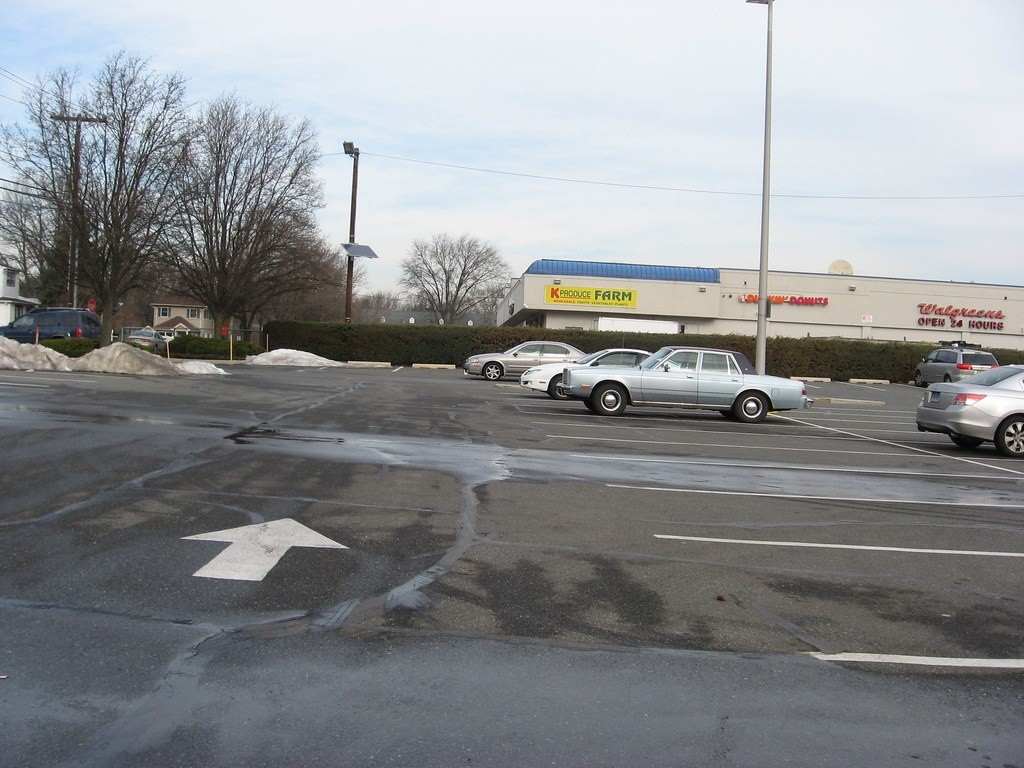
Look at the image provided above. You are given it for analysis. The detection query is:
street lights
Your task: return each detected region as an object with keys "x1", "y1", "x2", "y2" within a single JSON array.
[
  {"x1": 745, "y1": 0, "x2": 778, "y2": 376},
  {"x1": 342, "y1": 139, "x2": 360, "y2": 325}
]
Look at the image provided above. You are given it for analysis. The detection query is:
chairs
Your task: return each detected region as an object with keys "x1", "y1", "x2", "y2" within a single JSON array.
[{"x1": 681, "y1": 361, "x2": 689, "y2": 368}]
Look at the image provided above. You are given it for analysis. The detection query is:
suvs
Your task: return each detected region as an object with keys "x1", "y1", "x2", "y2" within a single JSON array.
[
  {"x1": 913, "y1": 347, "x2": 1001, "y2": 388},
  {"x1": 0, "y1": 307, "x2": 103, "y2": 345}
]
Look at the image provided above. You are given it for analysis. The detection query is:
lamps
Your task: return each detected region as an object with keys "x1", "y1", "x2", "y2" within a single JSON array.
[
  {"x1": 849, "y1": 285, "x2": 855, "y2": 291},
  {"x1": 553, "y1": 280, "x2": 560, "y2": 284},
  {"x1": 699, "y1": 287, "x2": 706, "y2": 292}
]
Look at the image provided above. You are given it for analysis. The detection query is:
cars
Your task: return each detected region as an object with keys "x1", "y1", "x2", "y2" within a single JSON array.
[
  {"x1": 519, "y1": 348, "x2": 689, "y2": 400},
  {"x1": 126, "y1": 330, "x2": 167, "y2": 352},
  {"x1": 560, "y1": 345, "x2": 815, "y2": 423},
  {"x1": 462, "y1": 340, "x2": 601, "y2": 381},
  {"x1": 915, "y1": 364, "x2": 1024, "y2": 458}
]
